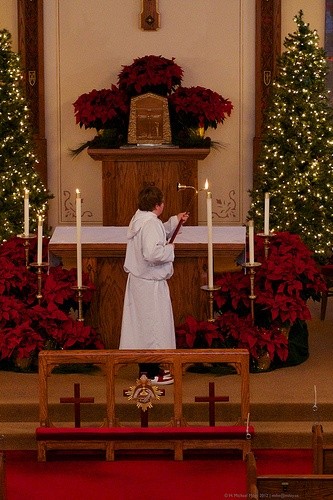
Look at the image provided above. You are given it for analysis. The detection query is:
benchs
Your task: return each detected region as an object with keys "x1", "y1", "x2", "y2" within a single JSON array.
[{"x1": 35, "y1": 425, "x2": 254, "y2": 463}]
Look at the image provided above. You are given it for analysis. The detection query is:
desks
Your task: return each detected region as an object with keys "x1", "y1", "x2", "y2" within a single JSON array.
[{"x1": 48, "y1": 226, "x2": 246, "y2": 349}]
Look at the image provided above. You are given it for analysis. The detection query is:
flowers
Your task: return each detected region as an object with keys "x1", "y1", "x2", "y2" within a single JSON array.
[
  {"x1": 0, "y1": 233, "x2": 105, "y2": 360},
  {"x1": 174, "y1": 230, "x2": 327, "y2": 362},
  {"x1": 72, "y1": 55, "x2": 234, "y2": 132}
]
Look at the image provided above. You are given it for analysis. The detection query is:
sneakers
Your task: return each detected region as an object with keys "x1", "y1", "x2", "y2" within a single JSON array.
[
  {"x1": 158, "y1": 369, "x2": 171, "y2": 376},
  {"x1": 150, "y1": 375, "x2": 174, "y2": 384}
]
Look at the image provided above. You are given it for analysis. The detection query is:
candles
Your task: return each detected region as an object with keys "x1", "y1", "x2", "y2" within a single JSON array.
[
  {"x1": 37, "y1": 221, "x2": 42, "y2": 265},
  {"x1": 76, "y1": 193, "x2": 82, "y2": 288},
  {"x1": 24, "y1": 194, "x2": 29, "y2": 237},
  {"x1": 264, "y1": 192, "x2": 269, "y2": 236},
  {"x1": 207, "y1": 191, "x2": 213, "y2": 288},
  {"x1": 249, "y1": 220, "x2": 254, "y2": 265}
]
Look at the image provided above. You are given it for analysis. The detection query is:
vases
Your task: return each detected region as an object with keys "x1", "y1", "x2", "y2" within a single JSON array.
[
  {"x1": 254, "y1": 351, "x2": 272, "y2": 371},
  {"x1": 13, "y1": 350, "x2": 34, "y2": 373}
]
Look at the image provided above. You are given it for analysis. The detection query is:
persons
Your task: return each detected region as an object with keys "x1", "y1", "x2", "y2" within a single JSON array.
[{"x1": 119, "y1": 187, "x2": 190, "y2": 385}]
